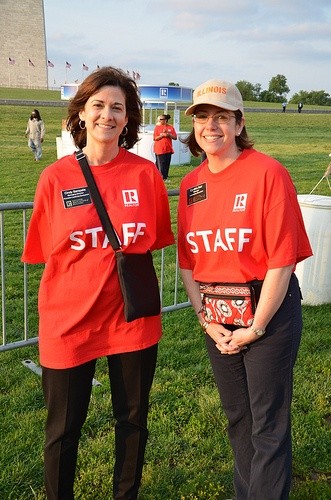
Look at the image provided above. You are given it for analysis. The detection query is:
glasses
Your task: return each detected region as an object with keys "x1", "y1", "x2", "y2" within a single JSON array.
[{"x1": 193, "y1": 113, "x2": 237, "y2": 124}]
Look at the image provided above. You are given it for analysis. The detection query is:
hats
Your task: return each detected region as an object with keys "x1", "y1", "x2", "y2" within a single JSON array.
[{"x1": 185, "y1": 79, "x2": 245, "y2": 116}]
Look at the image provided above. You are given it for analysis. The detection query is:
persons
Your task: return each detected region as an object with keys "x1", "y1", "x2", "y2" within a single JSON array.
[
  {"x1": 282, "y1": 101, "x2": 286, "y2": 112},
  {"x1": 298, "y1": 101, "x2": 303, "y2": 113},
  {"x1": 153, "y1": 114, "x2": 177, "y2": 181},
  {"x1": 175, "y1": 79, "x2": 314, "y2": 500},
  {"x1": 21, "y1": 66, "x2": 176, "y2": 500},
  {"x1": 24, "y1": 109, "x2": 44, "y2": 162}
]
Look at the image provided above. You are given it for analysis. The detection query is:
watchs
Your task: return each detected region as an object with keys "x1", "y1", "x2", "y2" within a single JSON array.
[{"x1": 251, "y1": 327, "x2": 265, "y2": 336}]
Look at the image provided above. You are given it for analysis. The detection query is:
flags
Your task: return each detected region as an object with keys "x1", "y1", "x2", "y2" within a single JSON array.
[
  {"x1": 29, "y1": 59, "x2": 34, "y2": 66},
  {"x1": 48, "y1": 60, "x2": 54, "y2": 68},
  {"x1": 66, "y1": 62, "x2": 72, "y2": 69},
  {"x1": 127, "y1": 70, "x2": 141, "y2": 79},
  {"x1": 9, "y1": 58, "x2": 15, "y2": 65},
  {"x1": 83, "y1": 64, "x2": 88, "y2": 71}
]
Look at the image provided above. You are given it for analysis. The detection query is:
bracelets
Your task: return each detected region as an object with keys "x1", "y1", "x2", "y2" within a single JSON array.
[{"x1": 202, "y1": 322, "x2": 209, "y2": 333}]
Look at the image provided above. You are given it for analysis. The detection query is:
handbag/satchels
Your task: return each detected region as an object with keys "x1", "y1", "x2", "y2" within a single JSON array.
[
  {"x1": 116, "y1": 250, "x2": 160, "y2": 322},
  {"x1": 198, "y1": 281, "x2": 258, "y2": 327}
]
